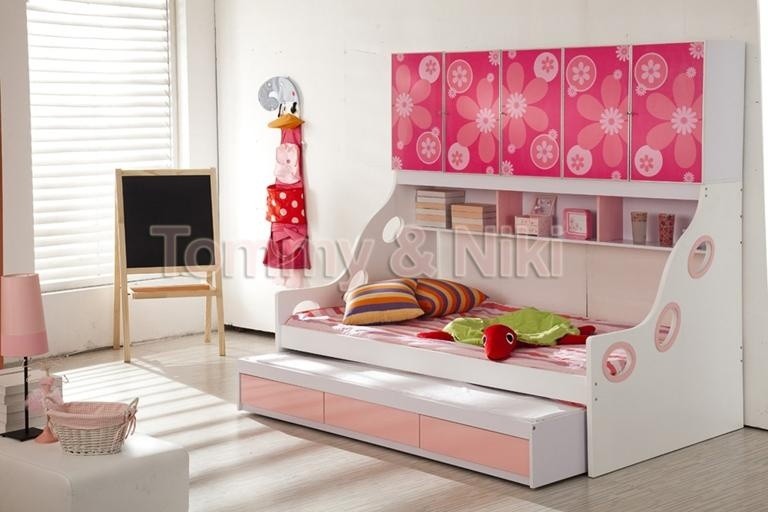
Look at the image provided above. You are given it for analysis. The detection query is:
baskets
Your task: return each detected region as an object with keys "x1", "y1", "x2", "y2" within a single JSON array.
[{"x1": 45, "y1": 397, "x2": 140, "y2": 454}]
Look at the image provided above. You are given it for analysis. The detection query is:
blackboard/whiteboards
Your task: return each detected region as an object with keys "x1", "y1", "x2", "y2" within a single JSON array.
[{"x1": 115, "y1": 168, "x2": 221, "y2": 274}]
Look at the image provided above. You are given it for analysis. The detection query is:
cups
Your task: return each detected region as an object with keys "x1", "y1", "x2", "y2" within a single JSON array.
[
  {"x1": 631, "y1": 211, "x2": 648, "y2": 243},
  {"x1": 658, "y1": 213, "x2": 676, "y2": 246}
]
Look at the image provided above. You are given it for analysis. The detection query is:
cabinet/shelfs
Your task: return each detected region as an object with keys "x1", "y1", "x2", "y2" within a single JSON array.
[{"x1": 390, "y1": 41, "x2": 744, "y2": 252}]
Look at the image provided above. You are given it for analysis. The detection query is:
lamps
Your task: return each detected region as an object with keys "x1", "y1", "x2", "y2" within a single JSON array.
[{"x1": 0, "y1": 270, "x2": 51, "y2": 442}]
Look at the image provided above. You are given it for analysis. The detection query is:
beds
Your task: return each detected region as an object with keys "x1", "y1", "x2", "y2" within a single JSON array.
[{"x1": 276, "y1": 283, "x2": 743, "y2": 477}]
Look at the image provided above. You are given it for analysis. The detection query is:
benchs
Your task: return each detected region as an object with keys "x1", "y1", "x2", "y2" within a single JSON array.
[{"x1": 236, "y1": 352, "x2": 585, "y2": 491}]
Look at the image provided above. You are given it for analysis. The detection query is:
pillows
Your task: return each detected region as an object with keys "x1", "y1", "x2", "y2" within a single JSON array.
[{"x1": 342, "y1": 273, "x2": 490, "y2": 326}]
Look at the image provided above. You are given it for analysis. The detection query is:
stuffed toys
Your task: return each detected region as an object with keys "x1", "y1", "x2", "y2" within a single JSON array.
[{"x1": 417, "y1": 307, "x2": 596, "y2": 361}]
